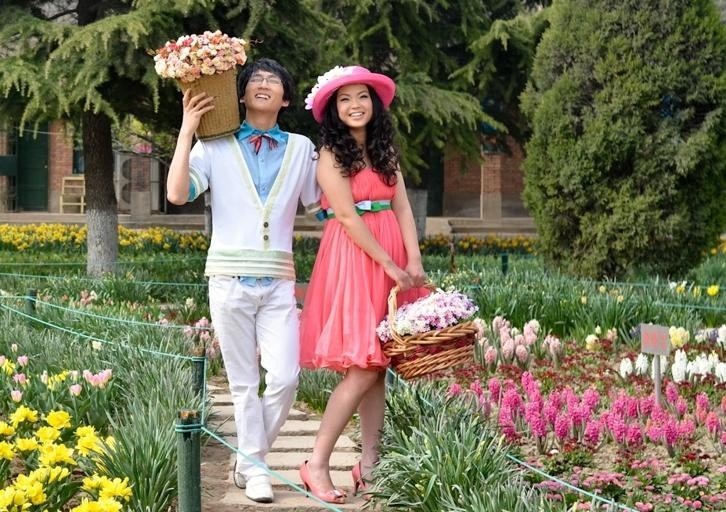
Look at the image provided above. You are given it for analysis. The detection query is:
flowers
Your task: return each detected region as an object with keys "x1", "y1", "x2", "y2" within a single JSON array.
[
  {"x1": 149, "y1": 27, "x2": 248, "y2": 85},
  {"x1": 374, "y1": 288, "x2": 481, "y2": 348}
]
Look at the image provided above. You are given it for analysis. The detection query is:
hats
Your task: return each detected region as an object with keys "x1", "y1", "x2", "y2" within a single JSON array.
[{"x1": 305, "y1": 65, "x2": 396, "y2": 123}]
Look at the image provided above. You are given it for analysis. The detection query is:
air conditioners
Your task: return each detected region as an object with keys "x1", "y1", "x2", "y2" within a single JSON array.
[{"x1": 115, "y1": 150, "x2": 160, "y2": 214}]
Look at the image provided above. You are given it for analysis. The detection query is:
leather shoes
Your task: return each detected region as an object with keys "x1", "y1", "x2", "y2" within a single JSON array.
[
  {"x1": 233, "y1": 461, "x2": 245, "y2": 489},
  {"x1": 245, "y1": 475, "x2": 273, "y2": 503}
]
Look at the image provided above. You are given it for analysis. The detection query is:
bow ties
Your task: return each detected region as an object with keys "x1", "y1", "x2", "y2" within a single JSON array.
[{"x1": 250, "y1": 133, "x2": 276, "y2": 154}]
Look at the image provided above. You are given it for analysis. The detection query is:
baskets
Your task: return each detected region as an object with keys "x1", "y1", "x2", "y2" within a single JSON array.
[
  {"x1": 382, "y1": 279, "x2": 478, "y2": 380},
  {"x1": 175, "y1": 67, "x2": 242, "y2": 142}
]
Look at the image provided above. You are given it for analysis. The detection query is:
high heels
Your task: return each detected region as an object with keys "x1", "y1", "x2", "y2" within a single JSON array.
[
  {"x1": 299, "y1": 460, "x2": 347, "y2": 504},
  {"x1": 351, "y1": 460, "x2": 370, "y2": 501}
]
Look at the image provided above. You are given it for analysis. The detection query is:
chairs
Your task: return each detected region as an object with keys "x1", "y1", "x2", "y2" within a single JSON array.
[{"x1": 59, "y1": 175, "x2": 86, "y2": 214}]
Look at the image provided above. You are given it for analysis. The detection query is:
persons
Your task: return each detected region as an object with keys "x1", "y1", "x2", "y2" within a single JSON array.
[
  {"x1": 300, "y1": 64, "x2": 427, "y2": 505},
  {"x1": 165, "y1": 58, "x2": 327, "y2": 502}
]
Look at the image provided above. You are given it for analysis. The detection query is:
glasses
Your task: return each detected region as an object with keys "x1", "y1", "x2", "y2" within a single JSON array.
[{"x1": 248, "y1": 76, "x2": 283, "y2": 88}]
